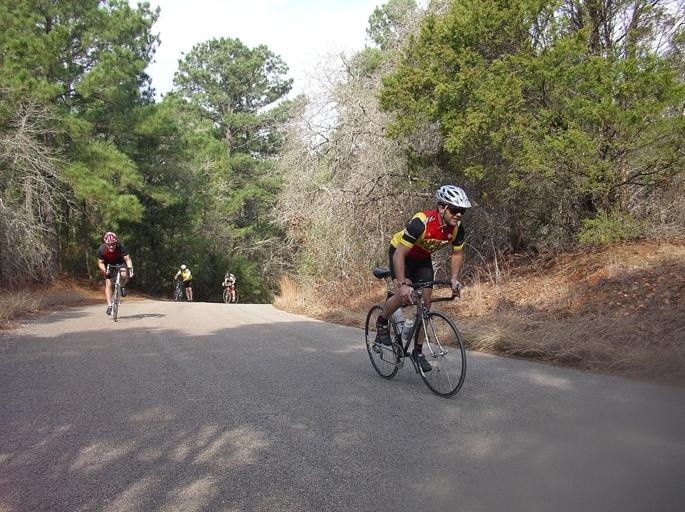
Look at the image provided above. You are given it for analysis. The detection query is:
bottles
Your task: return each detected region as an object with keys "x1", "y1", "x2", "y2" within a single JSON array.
[
  {"x1": 393, "y1": 308, "x2": 404, "y2": 335},
  {"x1": 402, "y1": 317, "x2": 414, "y2": 339}
]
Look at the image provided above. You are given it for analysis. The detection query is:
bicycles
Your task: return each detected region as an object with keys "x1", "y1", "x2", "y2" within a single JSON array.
[
  {"x1": 102, "y1": 262, "x2": 134, "y2": 324},
  {"x1": 362, "y1": 263, "x2": 470, "y2": 399},
  {"x1": 220, "y1": 281, "x2": 240, "y2": 304},
  {"x1": 170, "y1": 278, "x2": 184, "y2": 302}
]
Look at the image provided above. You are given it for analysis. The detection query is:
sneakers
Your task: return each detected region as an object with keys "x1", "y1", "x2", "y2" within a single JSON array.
[
  {"x1": 375, "y1": 318, "x2": 392, "y2": 346},
  {"x1": 106, "y1": 306, "x2": 112, "y2": 315},
  {"x1": 412, "y1": 348, "x2": 432, "y2": 372},
  {"x1": 120, "y1": 286, "x2": 126, "y2": 297}
]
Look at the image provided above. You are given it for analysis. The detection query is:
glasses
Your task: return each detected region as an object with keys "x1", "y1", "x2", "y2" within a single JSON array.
[{"x1": 447, "y1": 206, "x2": 467, "y2": 215}]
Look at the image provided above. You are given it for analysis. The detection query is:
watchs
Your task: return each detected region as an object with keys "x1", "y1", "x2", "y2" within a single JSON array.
[{"x1": 396, "y1": 281, "x2": 407, "y2": 289}]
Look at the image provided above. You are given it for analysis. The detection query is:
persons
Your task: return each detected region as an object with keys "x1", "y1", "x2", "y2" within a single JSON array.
[
  {"x1": 174, "y1": 263, "x2": 193, "y2": 302},
  {"x1": 221, "y1": 272, "x2": 236, "y2": 303},
  {"x1": 375, "y1": 184, "x2": 471, "y2": 373},
  {"x1": 96, "y1": 231, "x2": 135, "y2": 316}
]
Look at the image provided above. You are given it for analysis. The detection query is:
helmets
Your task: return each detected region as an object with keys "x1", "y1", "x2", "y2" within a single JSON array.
[
  {"x1": 103, "y1": 232, "x2": 117, "y2": 245},
  {"x1": 181, "y1": 264, "x2": 186, "y2": 270},
  {"x1": 435, "y1": 185, "x2": 472, "y2": 209}
]
